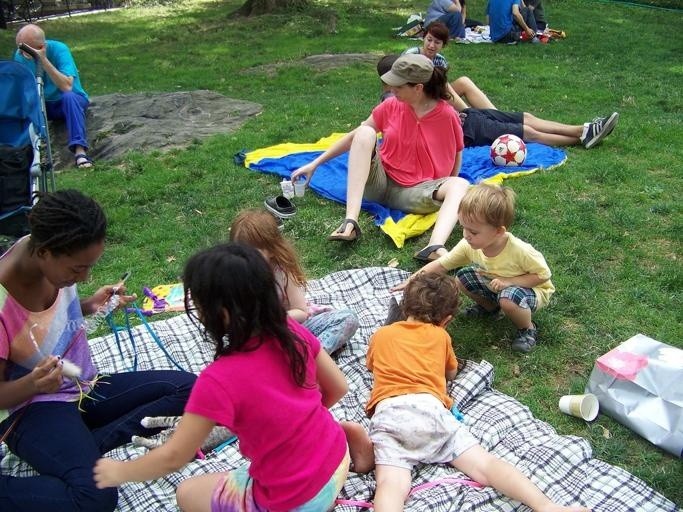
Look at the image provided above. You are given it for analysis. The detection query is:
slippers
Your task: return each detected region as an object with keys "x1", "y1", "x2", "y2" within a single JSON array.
[
  {"x1": 328, "y1": 219, "x2": 361, "y2": 240},
  {"x1": 413, "y1": 245, "x2": 449, "y2": 261}
]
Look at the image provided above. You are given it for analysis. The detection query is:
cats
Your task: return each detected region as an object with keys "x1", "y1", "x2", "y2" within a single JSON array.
[{"x1": 130, "y1": 416, "x2": 233, "y2": 454}]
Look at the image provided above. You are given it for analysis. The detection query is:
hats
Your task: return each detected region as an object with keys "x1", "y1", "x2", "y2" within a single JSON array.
[{"x1": 380, "y1": 54, "x2": 434, "y2": 87}]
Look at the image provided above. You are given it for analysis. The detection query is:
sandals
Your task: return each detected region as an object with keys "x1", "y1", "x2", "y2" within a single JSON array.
[{"x1": 73, "y1": 153, "x2": 94, "y2": 170}]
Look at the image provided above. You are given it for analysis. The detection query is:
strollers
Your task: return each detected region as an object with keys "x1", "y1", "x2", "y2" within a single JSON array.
[{"x1": 0, "y1": 42, "x2": 56, "y2": 238}]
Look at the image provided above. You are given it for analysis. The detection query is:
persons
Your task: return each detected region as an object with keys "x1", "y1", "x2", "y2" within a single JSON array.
[
  {"x1": 400, "y1": 21, "x2": 497, "y2": 113},
  {"x1": 92, "y1": 240, "x2": 375, "y2": 512},
  {"x1": 424, "y1": 0, "x2": 546, "y2": 45},
  {"x1": 14, "y1": 24, "x2": 93, "y2": 170},
  {"x1": 229, "y1": 208, "x2": 359, "y2": 355},
  {"x1": 0, "y1": 188, "x2": 198, "y2": 512},
  {"x1": 290, "y1": 55, "x2": 470, "y2": 262},
  {"x1": 390, "y1": 184, "x2": 556, "y2": 353},
  {"x1": 365, "y1": 270, "x2": 592, "y2": 512},
  {"x1": 377, "y1": 56, "x2": 619, "y2": 149}
]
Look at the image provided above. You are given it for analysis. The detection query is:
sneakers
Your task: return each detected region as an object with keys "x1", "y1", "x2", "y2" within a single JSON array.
[
  {"x1": 457, "y1": 305, "x2": 504, "y2": 324},
  {"x1": 579, "y1": 113, "x2": 618, "y2": 149},
  {"x1": 511, "y1": 321, "x2": 538, "y2": 353},
  {"x1": 264, "y1": 196, "x2": 296, "y2": 218}
]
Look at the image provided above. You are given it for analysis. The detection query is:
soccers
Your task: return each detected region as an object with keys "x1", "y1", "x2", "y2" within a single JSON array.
[{"x1": 491, "y1": 134, "x2": 526, "y2": 167}]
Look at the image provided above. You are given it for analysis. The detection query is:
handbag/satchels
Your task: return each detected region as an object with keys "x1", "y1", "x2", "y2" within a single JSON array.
[{"x1": 584, "y1": 334, "x2": 683, "y2": 457}]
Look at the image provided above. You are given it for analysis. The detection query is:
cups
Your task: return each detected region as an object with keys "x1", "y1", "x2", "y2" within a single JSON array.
[
  {"x1": 278, "y1": 180, "x2": 295, "y2": 197},
  {"x1": 558, "y1": 393, "x2": 602, "y2": 423},
  {"x1": 292, "y1": 181, "x2": 306, "y2": 197}
]
